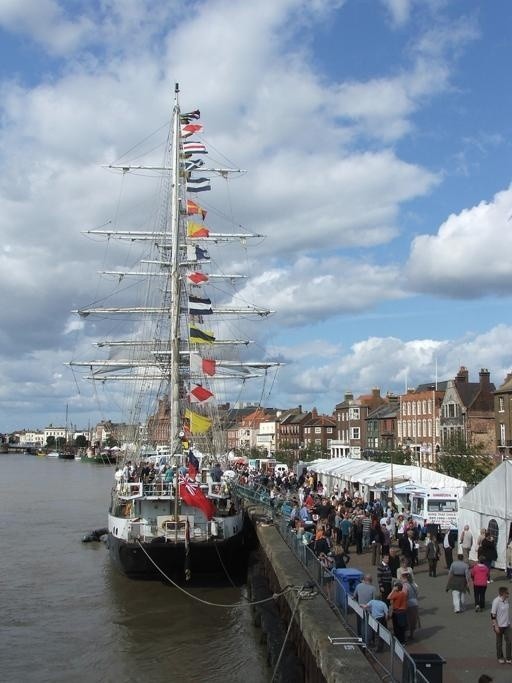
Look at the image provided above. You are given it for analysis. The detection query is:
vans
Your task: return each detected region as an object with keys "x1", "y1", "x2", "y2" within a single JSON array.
[{"x1": 408, "y1": 492, "x2": 458, "y2": 540}]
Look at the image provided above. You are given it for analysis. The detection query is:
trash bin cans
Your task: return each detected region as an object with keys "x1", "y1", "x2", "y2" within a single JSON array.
[
  {"x1": 403, "y1": 653, "x2": 446, "y2": 683},
  {"x1": 333, "y1": 568, "x2": 364, "y2": 614},
  {"x1": 427, "y1": 524, "x2": 441, "y2": 541}
]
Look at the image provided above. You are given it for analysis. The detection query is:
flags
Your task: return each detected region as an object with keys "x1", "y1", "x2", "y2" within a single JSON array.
[{"x1": 177, "y1": 106, "x2": 221, "y2": 522}]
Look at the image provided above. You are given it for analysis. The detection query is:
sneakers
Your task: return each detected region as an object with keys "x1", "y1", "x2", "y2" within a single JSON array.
[
  {"x1": 496, "y1": 657, "x2": 512, "y2": 664},
  {"x1": 474, "y1": 604, "x2": 480, "y2": 613}
]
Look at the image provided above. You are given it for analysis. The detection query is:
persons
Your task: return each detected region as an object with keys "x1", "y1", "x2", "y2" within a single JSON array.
[
  {"x1": 109, "y1": 449, "x2": 324, "y2": 496},
  {"x1": 277, "y1": 482, "x2": 511, "y2": 653},
  {"x1": 489, "y1": 585, "x2": 511, "y2": 665}
]
